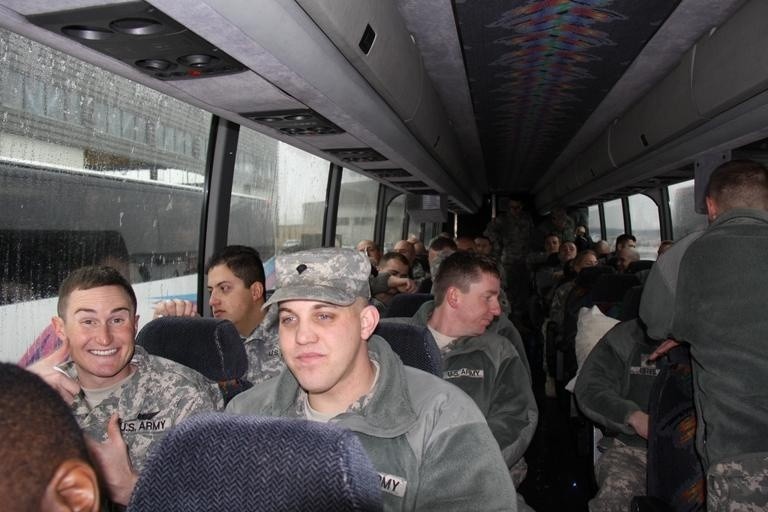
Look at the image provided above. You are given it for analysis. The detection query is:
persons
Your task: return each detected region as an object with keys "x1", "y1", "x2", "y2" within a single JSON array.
[{"x1": 2, "y1": 159, "x2": 767, "y2": 512}]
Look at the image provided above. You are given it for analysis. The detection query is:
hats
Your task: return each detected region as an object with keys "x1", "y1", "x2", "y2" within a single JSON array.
[{"x1": 257, "y1": 244, "x2": 373, "y2": 313}]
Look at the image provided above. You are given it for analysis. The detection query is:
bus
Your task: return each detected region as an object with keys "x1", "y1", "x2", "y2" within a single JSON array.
[{"x1": 0, "y1": 26, "x2": 278, "y2": 367}]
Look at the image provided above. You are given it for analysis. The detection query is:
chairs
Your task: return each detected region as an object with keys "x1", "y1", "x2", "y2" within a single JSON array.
[{"x1": 128, "y1": 245, "x2": 708, "y2": 512}]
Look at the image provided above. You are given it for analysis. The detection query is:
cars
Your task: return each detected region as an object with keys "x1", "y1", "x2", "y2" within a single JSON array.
[
  {"x1": 341, "y1": 241, "x2": 358, "y2": 252},
  {"x1": 590, "y1": 237, "x2": 661, "y2": 248},
  {"x1": 382, "y1": 240, "x2": 395, "y2": 253},
  {"x1": 282, "y1": 240, "x2": 308, "y2": 253}
]
